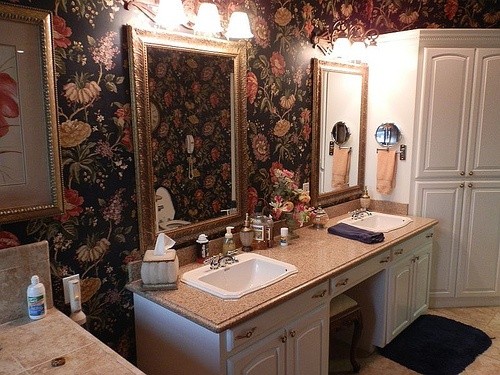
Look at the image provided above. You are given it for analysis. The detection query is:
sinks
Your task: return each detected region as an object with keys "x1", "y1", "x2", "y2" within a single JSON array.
[
  {"x1": 337, "y1": 211, "x2": 413, "y2": 233},
  {"x1": 179, "y1": 251, "x2": 299, "y2": 299}
]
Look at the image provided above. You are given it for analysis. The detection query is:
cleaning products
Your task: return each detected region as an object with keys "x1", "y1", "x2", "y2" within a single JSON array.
[
  {"x1": 195, "y1": 233, "x2": 210, "y2": 264},
  {"x1": 26, "y1": 275, "x2": 47, "y2": 320},
  {"x1": 221, "y1": 226, "x2": 236, "y2": 257}
]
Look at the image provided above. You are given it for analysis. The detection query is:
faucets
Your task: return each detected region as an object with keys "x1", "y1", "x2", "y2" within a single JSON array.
[
  {"x1": 348, "y1": 207, "x2": 372, "y2": 220},
  {"x1": 203, "y1": 248, "x2": 240, "y2": 271}
]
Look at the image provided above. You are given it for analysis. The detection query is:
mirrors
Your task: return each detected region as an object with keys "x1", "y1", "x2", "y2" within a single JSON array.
[
  {"x1": 127, "y1": 23, "x2": 249, "y2": 256},
  {"x1": 310, "y1": 58, "x2": 369, "y2": 209},
  {"x1": 375, "y1": 123, "x2": 405, "y2": 161},
  {"x1": 329, "y1": 121, "x2": 353, "y2": 156}
]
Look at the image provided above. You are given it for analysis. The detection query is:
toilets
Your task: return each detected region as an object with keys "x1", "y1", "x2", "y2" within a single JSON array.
[{"x1": 155, "y1": 186, "x2": 191, "y2": 232}]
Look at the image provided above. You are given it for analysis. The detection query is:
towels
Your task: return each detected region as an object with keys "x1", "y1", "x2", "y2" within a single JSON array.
[
  {"x1": 331, "y1": 148, "x2": 351, "y2": 190},
  {"x1": 375, "y1": 150, "x2": 398, "y2": 196},
  {"x1": 325, "y1": 222, "x2": 386, "y2": 245}
]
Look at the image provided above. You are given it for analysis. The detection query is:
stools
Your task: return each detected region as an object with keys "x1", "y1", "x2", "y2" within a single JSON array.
[{"x1": 329, "y1": 294, "x2": 363, "y2": 374}]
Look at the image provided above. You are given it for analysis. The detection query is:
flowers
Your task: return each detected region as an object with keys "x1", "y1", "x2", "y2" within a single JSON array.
[{"x1": 258, "y1": 162, "x2": 316, "y2": 226}]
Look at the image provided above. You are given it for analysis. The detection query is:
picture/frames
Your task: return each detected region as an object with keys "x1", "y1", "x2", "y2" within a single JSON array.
[{"x1": 0, "y1": 2, "x2": 66, "y2": 224}]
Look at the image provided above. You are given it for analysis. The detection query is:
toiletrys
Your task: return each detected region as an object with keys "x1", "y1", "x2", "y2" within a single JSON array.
[
  {"x1": 239, "y1": 212, "x2": 254, "y2": 251},
  {"x1": 359, "y1": 185, "x2": 371, "y2": 211},
  {"x1": 263, "y1": 209, "x2": 274, "y2": 248},
  {"x1": 250, "y1": 198, "x2": 267, "y2": 250}
]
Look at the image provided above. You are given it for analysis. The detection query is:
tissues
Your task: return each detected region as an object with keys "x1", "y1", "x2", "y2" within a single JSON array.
[{"x1": 139, "y1": 232, "x2": 180, "y2": 292}]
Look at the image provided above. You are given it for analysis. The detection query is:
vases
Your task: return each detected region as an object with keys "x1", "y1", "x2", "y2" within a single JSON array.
[{"x1": 284, "y1": 214, "x2": 299, "y2": 240}]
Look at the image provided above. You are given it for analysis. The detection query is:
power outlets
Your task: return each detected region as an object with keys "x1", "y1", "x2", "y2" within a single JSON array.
[{"x1": 61, "y1": 274, "x2": 81, "y2": 305}]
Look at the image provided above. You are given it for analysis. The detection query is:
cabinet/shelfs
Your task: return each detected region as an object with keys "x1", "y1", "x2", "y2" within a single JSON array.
[
  {"x1": 133, "y1": 226, "x2": 434, "y2": 375},
  {"x1": 367, "y1": 28, "x2": 500, "y2": 308}
]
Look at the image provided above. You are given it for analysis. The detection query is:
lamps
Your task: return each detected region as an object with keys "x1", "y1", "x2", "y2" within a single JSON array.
[
  {"x1": 312, "y1": 35, "x2": 367, "y2": 61},
  {"x1": 125, "y1": 0, "x2": 254, "y2": 39}
]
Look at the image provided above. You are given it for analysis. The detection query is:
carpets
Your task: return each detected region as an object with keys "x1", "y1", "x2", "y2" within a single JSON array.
[{"x1": 375, "y1": 315, "x2": 492, "y2": 375}]
